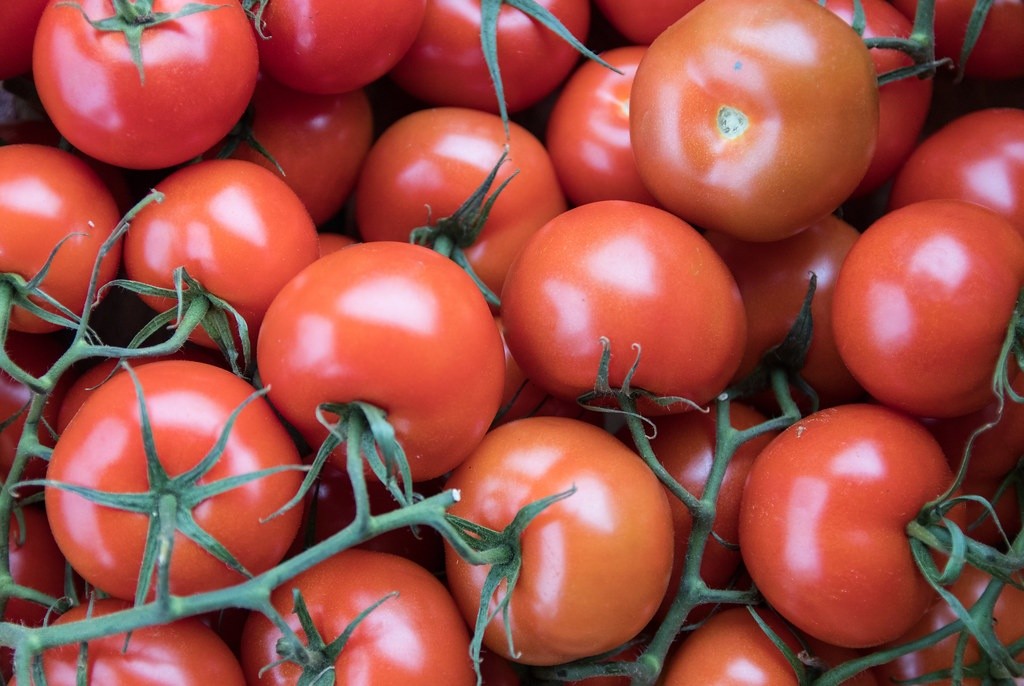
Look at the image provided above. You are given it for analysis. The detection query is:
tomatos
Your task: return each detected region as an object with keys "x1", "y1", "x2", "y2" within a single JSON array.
[{"x1": 0, "y1": 0, "x2": 1024, "y2": 686}]
